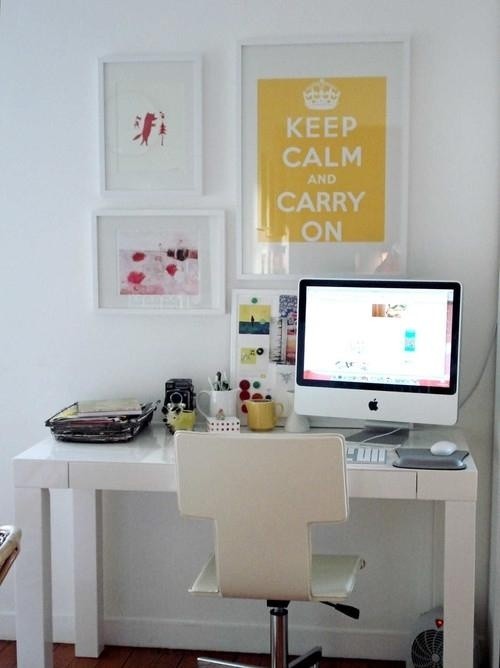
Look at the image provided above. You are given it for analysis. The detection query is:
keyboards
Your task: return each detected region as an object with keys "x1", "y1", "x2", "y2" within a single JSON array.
[{"x1": 346, "y1": 447, "x2": 388, "y2": 464}]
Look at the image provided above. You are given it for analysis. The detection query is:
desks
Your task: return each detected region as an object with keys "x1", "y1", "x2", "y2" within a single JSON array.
[{"x1": 13, "y1": 420, "x2": 480, "y2": 666}]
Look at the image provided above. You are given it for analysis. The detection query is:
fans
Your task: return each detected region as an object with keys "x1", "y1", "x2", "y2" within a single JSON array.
[{"x1": 402, "y1": 601, "x2": 444, "y2": 667}]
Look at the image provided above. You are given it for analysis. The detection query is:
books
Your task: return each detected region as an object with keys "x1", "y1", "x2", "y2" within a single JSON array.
[{"x1": 50, "y1": 397, "x2": 160, "y2": 440}]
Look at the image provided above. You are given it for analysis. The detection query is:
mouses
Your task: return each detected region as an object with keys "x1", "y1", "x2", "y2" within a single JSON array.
[{"x1": 431, "y1": 440, "x2": 457, "y2": 456}]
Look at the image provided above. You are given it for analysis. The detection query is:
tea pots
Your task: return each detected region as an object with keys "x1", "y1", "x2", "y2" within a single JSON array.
[
  {"x1": 195, "y1": 387, "x2": 242, "y2": 419},
  {"x1": 244, "y1": 398, "x2": 283, "y2": 431}
]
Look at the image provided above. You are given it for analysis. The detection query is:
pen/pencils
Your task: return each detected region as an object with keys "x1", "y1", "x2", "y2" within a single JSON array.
[{"x1": 207, "y1": 371, "x2": 232, "y2": 391}]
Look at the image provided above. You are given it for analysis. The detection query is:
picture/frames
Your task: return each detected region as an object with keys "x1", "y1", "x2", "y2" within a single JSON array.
[
  {"x1": 98, "y1": 58, "x2": 204, "y2": 202},
  {"x1": 87, "y1": 206, "x2": 225, "y2": 318}
]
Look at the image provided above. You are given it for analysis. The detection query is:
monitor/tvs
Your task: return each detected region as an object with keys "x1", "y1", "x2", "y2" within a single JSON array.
[{"x1": 297, "y1": 278, "x2": 462, "y2": 449}]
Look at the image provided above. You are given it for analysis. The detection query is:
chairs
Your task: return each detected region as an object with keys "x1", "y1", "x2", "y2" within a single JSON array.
[{"x1": 170, "y1": 426, "x2": 371, "y2": 666}]
